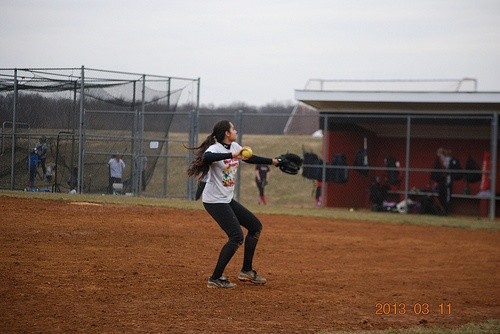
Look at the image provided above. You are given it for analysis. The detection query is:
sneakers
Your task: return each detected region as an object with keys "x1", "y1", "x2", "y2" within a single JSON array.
[
  {"x1": 207, "y1": 277, "x2": 235, "y2": 287},
  {"x1": 238, "y1": 272, "x2": 267, "y2": 285}
]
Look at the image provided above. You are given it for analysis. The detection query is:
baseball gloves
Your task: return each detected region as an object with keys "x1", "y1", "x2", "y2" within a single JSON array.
[{"x1": 274, "y1": 153, "x2": 303, "y2": 175}]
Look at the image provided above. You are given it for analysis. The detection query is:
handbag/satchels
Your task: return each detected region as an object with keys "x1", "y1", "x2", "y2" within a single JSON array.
[{"x1": 354, "y1": 150, "x2": 482, "y2": 212}]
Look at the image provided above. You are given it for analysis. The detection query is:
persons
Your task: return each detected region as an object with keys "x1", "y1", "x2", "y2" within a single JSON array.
[
  {"x1": 26, "y1": 136, "x2": 56, "y2": 190},
  {"x1": 311, "y1": 178, "x2": 323, "y2": 206},
  {"x1": 107, "y1": 152, "x2": 125, "y2": 193},
  {"x1": 369, "y1": 176, "x2": 393, "y2": 212},
  {"x1": 68, "y1": 168, "x2": 78, "y2": 191},
  {"x1": 195, "y1": 173, "x2": 209, "y2": 200},
  {"x1": 432, "y1": 147, "x2": 459, "y2": 204},
  {"x1": 255, "y1": 164, "x2": 270, "y2": 204},
  {"x1": 185, "y1": 119, "x2": 303, "y2": 288}
]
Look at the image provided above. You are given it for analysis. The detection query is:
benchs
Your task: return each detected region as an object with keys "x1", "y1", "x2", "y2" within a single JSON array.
[{"x1": 386, "y1": 189, "x2": 500, "y2": 215}]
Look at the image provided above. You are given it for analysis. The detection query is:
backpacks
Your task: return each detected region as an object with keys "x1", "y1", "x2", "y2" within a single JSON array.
[
  {"x1": 299, "y1": 152, "x2": 321, "y2": 179},
  {"x1": 330, "y1": 157, "x2": 348, "y2": 184}
]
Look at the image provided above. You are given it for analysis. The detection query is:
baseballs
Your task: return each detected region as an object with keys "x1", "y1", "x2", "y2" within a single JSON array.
[{"x1": 242, "y1": 147, "x2": 253, "y2": 158}]
